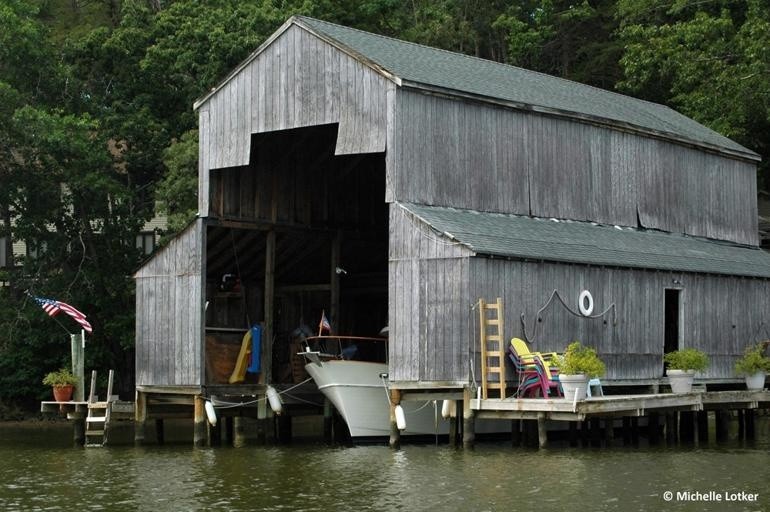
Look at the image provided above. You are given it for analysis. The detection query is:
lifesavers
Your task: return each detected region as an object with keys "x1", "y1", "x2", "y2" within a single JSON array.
[{"x1": 578, "y1": 289, "x2": 595, "y2": 315}]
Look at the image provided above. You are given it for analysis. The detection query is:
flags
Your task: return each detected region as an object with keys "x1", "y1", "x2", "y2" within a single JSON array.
[{"x1": 27, "y1": 293, "x2": 94, "y2": 335}]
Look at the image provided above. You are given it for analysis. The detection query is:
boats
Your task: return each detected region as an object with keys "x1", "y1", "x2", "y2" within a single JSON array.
[{"x1": 296, "y1": 307, "x2": 693, "y2": 439}]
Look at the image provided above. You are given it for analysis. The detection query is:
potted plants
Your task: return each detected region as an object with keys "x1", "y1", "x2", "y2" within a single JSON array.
[
  {"x1": 664, "y1": 349, "x2": 709, "y2": 396},
  {"x1": 733, "y1": 344, "x2": 770, "y2": 390},
  {"x1": 553, "y1": 341, "x2": 605, "y2": 399},
  {"x1": 43, "y1": 368, "x2": 78, "y2": 402}
]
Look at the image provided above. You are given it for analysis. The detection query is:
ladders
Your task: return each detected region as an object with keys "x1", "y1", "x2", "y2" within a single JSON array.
[
  {"x1": 83, "y1": 370, "x2": 114, "y2": 448},
  {"x1": 479, "y1": 298, "x2": 507, "y2": 400}
]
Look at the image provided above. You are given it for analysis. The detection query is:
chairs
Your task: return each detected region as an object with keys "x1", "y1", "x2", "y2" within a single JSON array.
[{"x1": 508, "y1": 338, "x2": 563, "y2": 398}]
